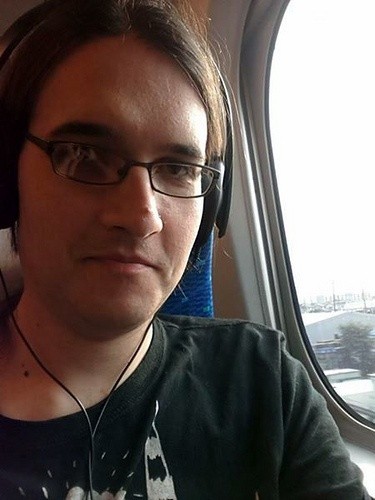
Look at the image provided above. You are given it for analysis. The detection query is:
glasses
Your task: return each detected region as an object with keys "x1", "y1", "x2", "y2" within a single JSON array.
[{"x1": 21, "y1": 132, "x2": 222, "y2": 200}]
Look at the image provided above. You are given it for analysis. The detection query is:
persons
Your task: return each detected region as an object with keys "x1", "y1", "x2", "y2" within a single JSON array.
[{"x1": 0, "y1": 0, "x2": 375, "y2": 500}]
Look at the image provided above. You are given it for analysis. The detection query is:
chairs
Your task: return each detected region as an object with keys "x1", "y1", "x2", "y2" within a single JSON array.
[{"x1": 0, "y1": 227, "x2": 214, "y2": 316}]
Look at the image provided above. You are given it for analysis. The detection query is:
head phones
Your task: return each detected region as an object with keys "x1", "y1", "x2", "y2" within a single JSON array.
[{"x1": 0, "y1": 0, "x2": 233, "y2": 250}]
